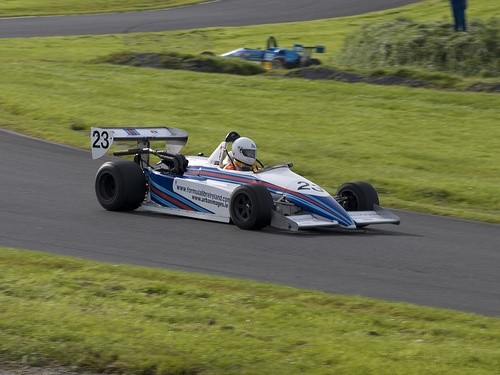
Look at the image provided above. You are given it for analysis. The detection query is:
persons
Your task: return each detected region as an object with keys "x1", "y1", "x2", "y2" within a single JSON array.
[
  {"x1": 223, "y1": 137, "x2": 260, "y2": 172},
  {"x1": 448, "y1": 0, "x2": 467, "y2": 33}
]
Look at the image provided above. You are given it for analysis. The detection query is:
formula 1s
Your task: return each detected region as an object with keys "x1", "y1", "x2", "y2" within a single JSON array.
[
  {"x1": 199, "y1": 35, "x2": 328, "y2": 70},
  {"x1": 90, "y1": 125, "x2": 400, "y2": 235}
]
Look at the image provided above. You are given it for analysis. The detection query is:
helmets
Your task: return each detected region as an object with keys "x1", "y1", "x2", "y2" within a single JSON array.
[{"x1": 232, "y1": 136, "x2": 256, "y2": 166}]
